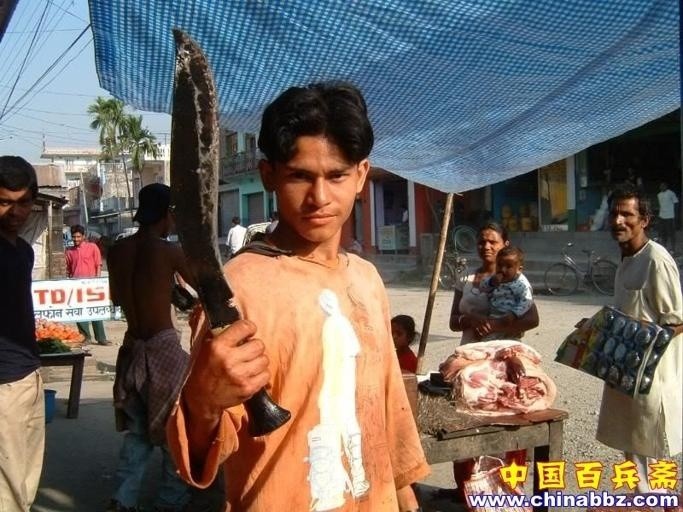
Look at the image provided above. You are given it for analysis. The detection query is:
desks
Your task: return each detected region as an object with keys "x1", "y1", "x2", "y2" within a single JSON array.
[
  {"x1": 416, "y1": 407, "x2": 572, "y2": 512},
  {"x1": 38, "y1": 347, "x2": 92, "y2": 418}
]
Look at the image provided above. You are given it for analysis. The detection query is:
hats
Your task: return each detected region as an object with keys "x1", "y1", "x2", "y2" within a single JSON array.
[{"x1": 133, "y1": 183, "x2": 170, "y2": 226}]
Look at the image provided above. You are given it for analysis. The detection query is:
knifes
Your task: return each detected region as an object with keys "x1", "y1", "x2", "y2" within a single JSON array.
[{"x1": 169, "y1": 26, "x2": 295, "y2": 442}]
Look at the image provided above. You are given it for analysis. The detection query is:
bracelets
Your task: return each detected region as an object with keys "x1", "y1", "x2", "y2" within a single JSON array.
[{"x1": 456, "y1": 314, "x2": 467, "y2": 323}]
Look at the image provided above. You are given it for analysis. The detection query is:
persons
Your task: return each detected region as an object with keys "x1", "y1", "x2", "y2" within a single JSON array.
[
  {"x1": 398, "y1": 205, "x2": 409, "y2": 225},
  {"x1": 163, "y1": 81, "x2": 433, "y2": 512},
  {"x1": 64, "y1": 224, "x2": 112, "y2": 346},
  {"x1": 0, "y1": 155, "x2": 47, "y2": 512},
  {"x1": 654, "y1": 181, "x2": 680, "y2": 256},
  {"x1": 221, "y1": 217, "x2": 246, "y2": 260},
  {"x1": 478, "y1": 244, "x2": 533, "y2": 344},
  {"x1": 447, "y1": 221, "x2": 540, "y2": 348},
  {"x1": 625, "y1": 166, "x2": 644, "y2": 194},
  {"x1": 105, "y1": 182, "x2": 198, "y2": 511},
  {"x1": 263, "y1": 211, "x2": 280, "y2": 234},
  {"x1": 572, "y1": 180, "x2": 683, "y2": 512},
  {"x1": 345, "y1": 236, "x2": 362, "y2": 256},
  {"x1": 387, "y1": 314, "x2": 419, "y2": 374}
]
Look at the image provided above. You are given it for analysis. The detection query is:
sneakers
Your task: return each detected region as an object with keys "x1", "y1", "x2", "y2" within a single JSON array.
[{"x1": 83, "y1": 337, "x2": 112, "y2": 345}]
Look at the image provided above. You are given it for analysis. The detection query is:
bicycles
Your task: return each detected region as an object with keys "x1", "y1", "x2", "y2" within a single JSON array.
[
  {"x1": 543, "y1": 242, "x2": 619, "y2": 297},
  {"x1": 438, "y1": 249, "x2": 470, "y2": 291}
]
